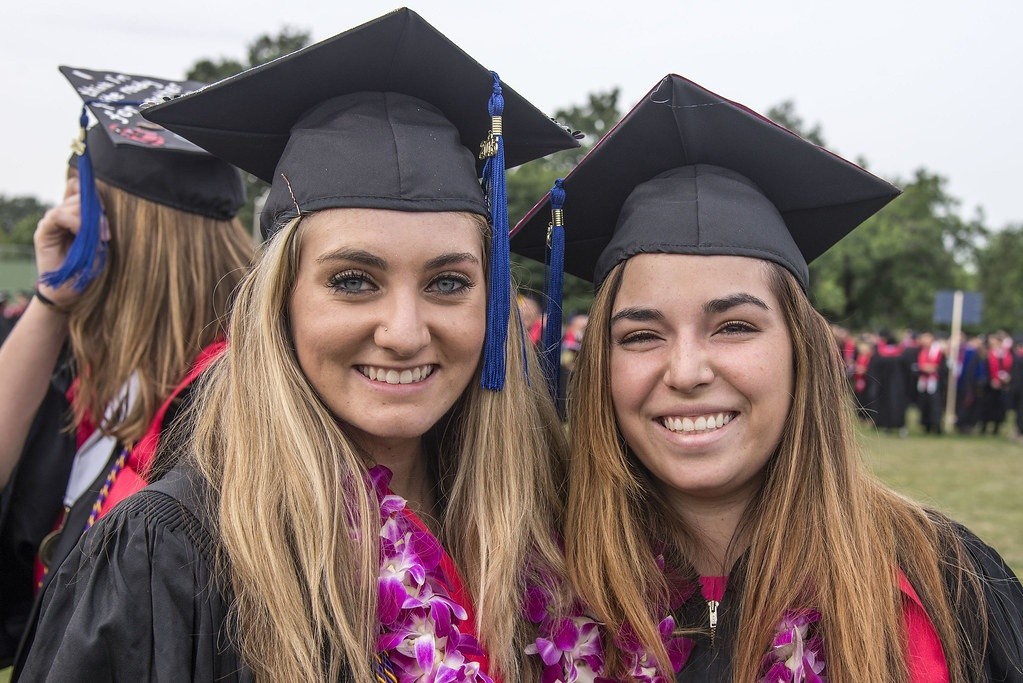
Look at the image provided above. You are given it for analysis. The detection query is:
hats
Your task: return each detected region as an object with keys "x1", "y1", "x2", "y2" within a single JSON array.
[
  {"x1": 40, "y1": 64, "x2": 246, "y2": 292},
  {"x1": 139, "y1": 6, "x2": 585, "y2": 392},
  {"x1": 508, "y1": 73, "x2": 904, "y2": 417}
]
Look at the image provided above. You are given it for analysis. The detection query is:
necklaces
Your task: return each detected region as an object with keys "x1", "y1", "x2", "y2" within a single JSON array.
[
  {"x1": 611, "y1": 530, "x2": 827, "y2": 683},
  {"x1": 359, "y1": 463, "x2": 604, "y2": 683}
]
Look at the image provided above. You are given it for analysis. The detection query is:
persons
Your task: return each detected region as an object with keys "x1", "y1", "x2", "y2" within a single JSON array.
[
  {"x1": 516, "y1": 280, "x2": 590, "y2": 422},
  {"x1": 507, "y1": 73, "x2": 1023, "y2": 683},
  {"x1": 827, "y1": 316, "x2": 1023, "y2": 436},
  {"x1": 21, "y1": 6, "x2": 581, "y2": 683},
  {"x1": 0, "y1": 66, "x2": 262, "y2": 671}
]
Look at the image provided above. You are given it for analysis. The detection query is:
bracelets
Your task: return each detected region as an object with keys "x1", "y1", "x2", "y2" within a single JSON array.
[{"x1": 36, "y1": 290, "x2": 71, "y2": 314}]
